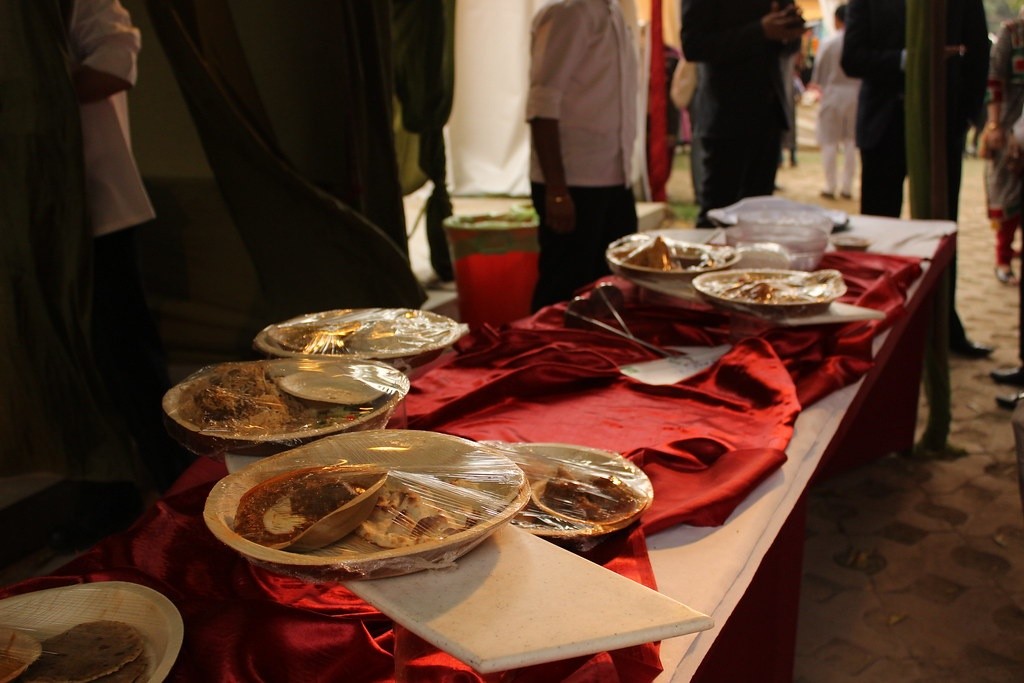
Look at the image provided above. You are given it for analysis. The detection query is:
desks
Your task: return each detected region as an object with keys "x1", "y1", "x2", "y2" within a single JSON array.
[{"x1": 0, "y1": 212, "x2": 963, "y2": 683}]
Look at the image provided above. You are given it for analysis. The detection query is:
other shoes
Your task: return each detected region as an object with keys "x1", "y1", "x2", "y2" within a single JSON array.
[
  {"x1": 991, "y1": 368, "x2": 1024, "y2": 387},
  {"x1": 996, "y1": 393, "x2": 1024, "y2": 412},
  {"x1": 948, "y1": 342, "x2": 991, "y2": 360},
  {"x1": 995, "y1": 268, "x2": 1016, "y2": 284}
]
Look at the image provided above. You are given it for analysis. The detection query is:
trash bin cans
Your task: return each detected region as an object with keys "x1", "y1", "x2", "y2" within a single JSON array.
[{"x1": 441, "y1": 210, "x2": 539, "y2": 327}]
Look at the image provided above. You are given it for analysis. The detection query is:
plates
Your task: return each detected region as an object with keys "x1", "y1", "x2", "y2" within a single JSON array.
[
  {"x1": 203, "y1": 428, "x2": 532, "y2": 584},
  {"x1": 690, "y1": 267, "x2": 847, "y2": 312},
  {"x1": 501, "y1": 442, "x2": 654, "y2": 537},
  {"x1": 0, "y1": 580, "x2": 184, "y2": 683},
  {"x1": 162, "y1": 356, "x2": 411, "y2": 443},
  {"x1": 253, "y1": 307, "x2": 462, "y2": 359},
  {"x1": 606, "y1": 238, "x2": 742, "y2": 274}
]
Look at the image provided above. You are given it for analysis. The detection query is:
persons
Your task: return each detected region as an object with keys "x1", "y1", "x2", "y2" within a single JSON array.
[
  {"x1": 836, "y1": 0, "x2": 994, "y2": 364},
  {"x1": 810, "y1": 5, "x2": 865, "y2": 199},
  {"x1": 977, "y1": 5, "x2": 1024, "y2": 487},
  {"x1": 68, "y1": 0, "x2": 173, "y2": 488},
  {"x1": 677, "y1": 0, "x2": 808, "y2": 235},
  {"x1": 782, "y1": 68, "x2": 808, "y2": 168},
  {"x1": 522, "y1": 0, "x2": 650, "y2": 318}
]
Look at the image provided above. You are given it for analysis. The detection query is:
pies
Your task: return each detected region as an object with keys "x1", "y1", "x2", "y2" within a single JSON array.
[
  {"x1": 356, "y1": 476, "x2": 483, "y2": 548},
  {"x1": 0, "y1": 620, "x2": 148, "y2": 683}
]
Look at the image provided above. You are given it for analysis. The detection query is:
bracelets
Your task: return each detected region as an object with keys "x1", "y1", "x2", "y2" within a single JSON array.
[{"x1": 546, "y1": 196, "x2": 570, "y2": 204}]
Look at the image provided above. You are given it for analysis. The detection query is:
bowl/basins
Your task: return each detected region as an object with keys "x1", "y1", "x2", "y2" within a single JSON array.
[
  {"x1": 832, "y1": 237, "x2": 872, "y2": 251},
  {"x1": 725, "y1": 224, "x2": 828, "y2": 272}
]
